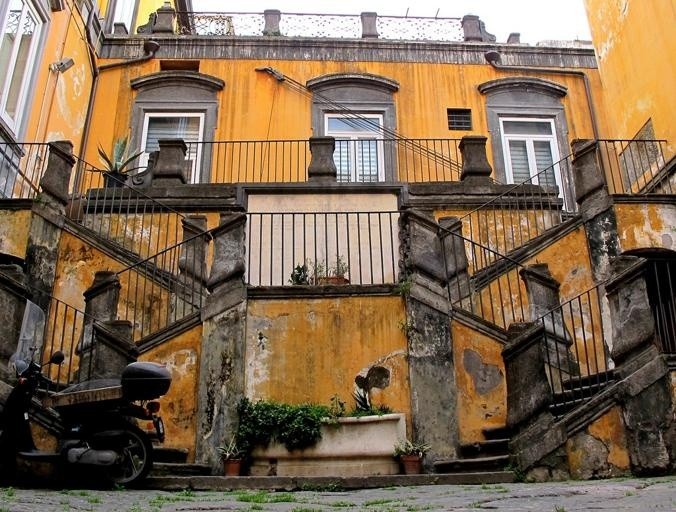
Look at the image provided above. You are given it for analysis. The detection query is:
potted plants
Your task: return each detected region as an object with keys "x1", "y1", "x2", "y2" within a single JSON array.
[
  {"x1": 85, "y1": 134, "x2": 148, "y2": 189},
  {"x1": 288, "y1": 254, "x2": 349, "y2": 286},
  {"x1": 215, "y1": 432, "x2": 247, "y2": 476},
  {"x1": 392, "y1": 437, "x2": 433, "y2": 475}
]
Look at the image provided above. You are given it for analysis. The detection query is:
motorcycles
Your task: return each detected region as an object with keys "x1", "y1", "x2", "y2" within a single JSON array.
[{"x1": 0, "y1": 346, "x2": 173, "y2": 490}]
